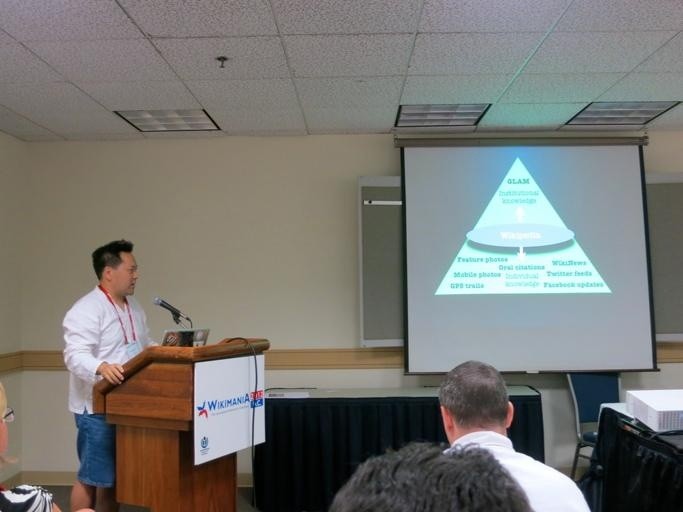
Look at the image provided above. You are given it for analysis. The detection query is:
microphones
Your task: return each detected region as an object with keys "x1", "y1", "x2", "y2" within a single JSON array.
[{"x1": 153, "y1": 297, "x2": 179, "y2": 317}]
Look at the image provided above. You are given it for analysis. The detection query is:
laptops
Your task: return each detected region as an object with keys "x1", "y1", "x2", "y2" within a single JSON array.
[{"x1": 160, "y1": 328, "x2": 210, "y2": 346}]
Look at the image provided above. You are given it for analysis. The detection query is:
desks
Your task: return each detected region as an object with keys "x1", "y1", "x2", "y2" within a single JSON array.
[
  {"x1": 253, "y1": 388, "x2": 545, "y2": 512},
  {"x1": 585, "y1": 401, "x2": 683, "y2": 511}
]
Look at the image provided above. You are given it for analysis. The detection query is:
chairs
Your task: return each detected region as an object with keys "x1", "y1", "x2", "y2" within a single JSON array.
[{"x1": 567, "y1": 372, "x2": 623, "y2": 479}]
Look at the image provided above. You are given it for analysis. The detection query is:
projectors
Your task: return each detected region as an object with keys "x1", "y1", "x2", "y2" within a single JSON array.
[{"x1": 625, "y1": 389, "x2": 683, "y2": 433}]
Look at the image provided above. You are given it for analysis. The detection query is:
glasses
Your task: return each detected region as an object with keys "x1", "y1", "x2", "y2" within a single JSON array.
[{"x1": 4, "y1": 406, "x2": 15, "y2": 423}]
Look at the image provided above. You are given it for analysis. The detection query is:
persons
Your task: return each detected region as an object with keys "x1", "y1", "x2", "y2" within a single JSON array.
[
  {"x1": 0, "y1": 381, "x2": 62, "y2": 512},
  {"x1": 437, "y1": 360, "x2": 592, "y2": 512},
  {"x1": 60, "y1": 238, "x2": 159, "y2": 512},
  {"x1": 328, "y1": 440, "x2": 536, "y2": 512}
]
